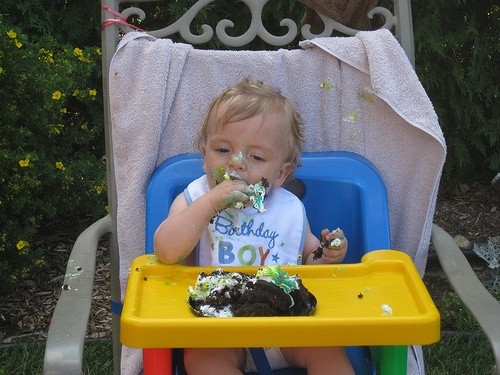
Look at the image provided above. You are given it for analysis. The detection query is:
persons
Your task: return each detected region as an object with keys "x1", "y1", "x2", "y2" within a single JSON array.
[{"x1": 153, "y1": 76, "x2": 357, "y2": 375}]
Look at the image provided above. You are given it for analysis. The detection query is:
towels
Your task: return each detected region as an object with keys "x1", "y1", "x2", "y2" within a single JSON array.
[{"x1": 109, "y1": 27, "x2": 448, "y2": 375}]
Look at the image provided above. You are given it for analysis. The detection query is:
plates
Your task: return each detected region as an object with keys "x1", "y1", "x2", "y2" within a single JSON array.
[{"x1": 189, "y1": 287, "x2": 317, "y2": 316}]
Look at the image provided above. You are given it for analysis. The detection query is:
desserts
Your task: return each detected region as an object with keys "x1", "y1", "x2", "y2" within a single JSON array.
[{"x1": 186, "y1": 265, "x2": 317, "y2": 318}]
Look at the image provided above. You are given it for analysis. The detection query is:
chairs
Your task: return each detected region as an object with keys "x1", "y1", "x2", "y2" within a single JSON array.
[{"x1": 39, "y1": 0, "x2": 500, "y2": 375}]
